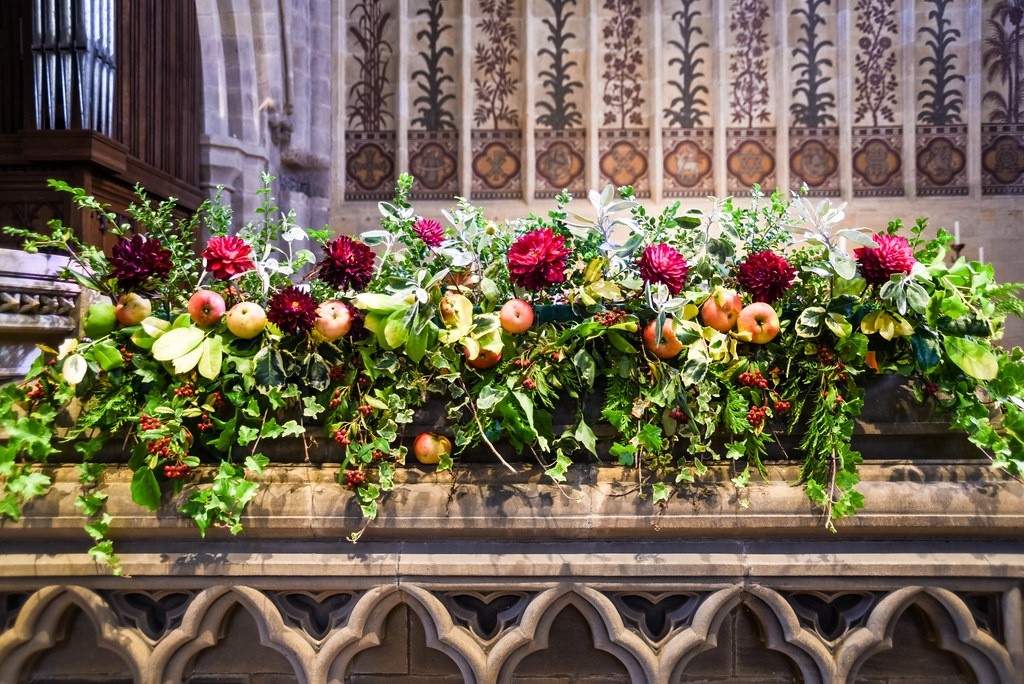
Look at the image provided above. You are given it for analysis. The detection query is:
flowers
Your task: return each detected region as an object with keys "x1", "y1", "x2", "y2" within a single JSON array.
[{"x1": 0, "y1": 172, "x2": 1024, "y2": 580}]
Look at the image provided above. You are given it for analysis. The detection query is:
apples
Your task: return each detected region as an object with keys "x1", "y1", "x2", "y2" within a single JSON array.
[
  {"x1": 414, "y1": 432, "x2": 451, "y2": 464},
  {"x1": 313, "y1": 299, "x2": 352, "y2": 339},
  {"x1": 642, "y1": 289, "x2": 780, "y2": 359},
  {"x1": 440, "y1": 294, "x2": 535, "y2": 369},
  {"x1": 187, "y1": 289, "x2": 226, "y2": 326},
  {"x1": 81, "y1": 303, "x2": 117, "y2": 338},
  {"x1": 114, "y1": 292, "x2": 151, "y2": 326},
  {"x1": 226, "y1": 302, "x2": 266, "y2": 339}
]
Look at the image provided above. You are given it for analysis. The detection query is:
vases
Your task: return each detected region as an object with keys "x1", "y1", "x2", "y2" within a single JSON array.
[
  {"x1": 464, "y1": 305, "x2": 604, "y2": 423},
  {"x1": 853, "y1": 374, "x2": 936, "y2": 422},
  {"x1": 411, "y1": 389, "x2": 455, "y2": 424}
]
